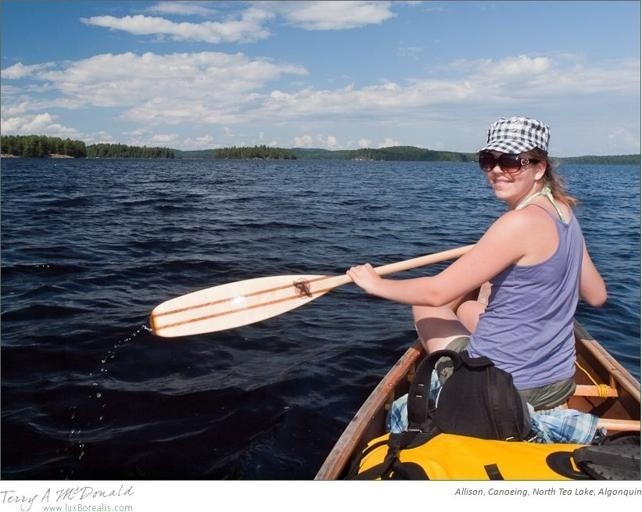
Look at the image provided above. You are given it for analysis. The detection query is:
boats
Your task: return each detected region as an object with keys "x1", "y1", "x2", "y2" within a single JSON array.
[{"x1": 314, "y1": 299, "x2": 640, "y2": 479}]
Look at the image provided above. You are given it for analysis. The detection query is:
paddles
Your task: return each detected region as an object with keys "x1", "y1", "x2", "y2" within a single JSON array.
[{"x1": 150, "y1": 239, "x2": 475, "y2": 340}]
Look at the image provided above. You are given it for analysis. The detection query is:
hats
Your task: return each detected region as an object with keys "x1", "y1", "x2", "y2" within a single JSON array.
[{"x1": 474, "y1": 115, "x2": 551, "y2": 155}]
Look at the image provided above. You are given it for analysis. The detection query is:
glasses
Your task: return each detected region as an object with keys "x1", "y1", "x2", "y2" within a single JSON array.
[{"x1": 478, "y1": 150, "x2": 540, "y2": 174}]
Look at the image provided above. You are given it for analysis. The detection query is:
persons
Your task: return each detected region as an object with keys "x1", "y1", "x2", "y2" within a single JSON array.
[{"x1": 344, "y1": 113, "x2": 611, "y2": 410}]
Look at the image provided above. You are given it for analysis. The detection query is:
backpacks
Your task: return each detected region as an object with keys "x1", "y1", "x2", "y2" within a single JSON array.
[{"x1": 404, "y1": 349, "x2": 533, "y2": 448}]
